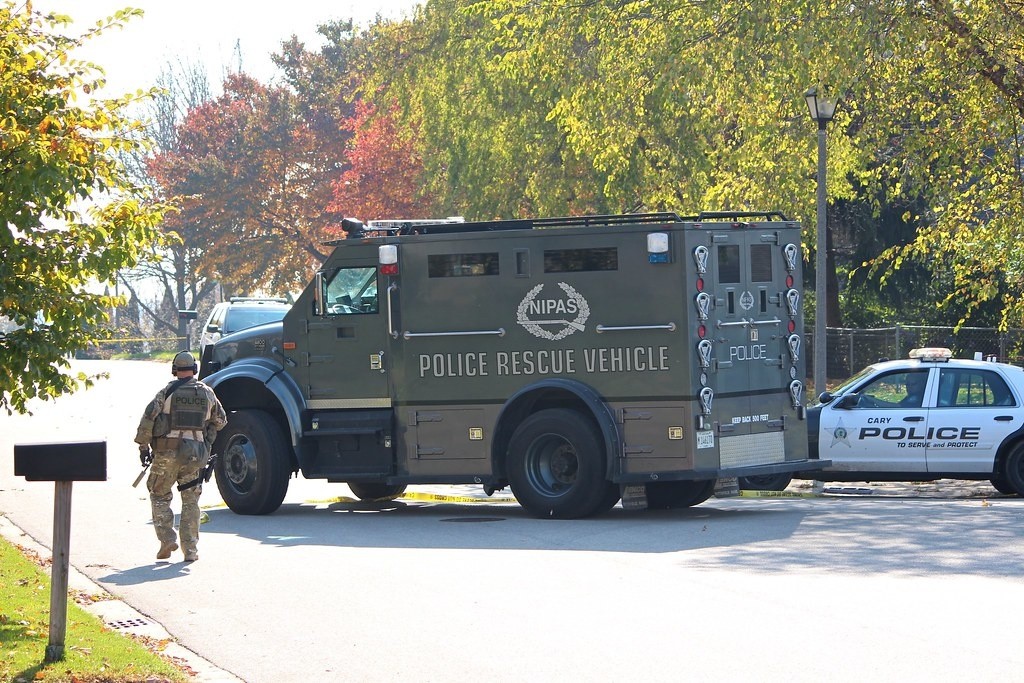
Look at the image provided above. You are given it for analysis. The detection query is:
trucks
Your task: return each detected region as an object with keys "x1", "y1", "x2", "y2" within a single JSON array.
[{"x1": 192, "y1": 211, "x2": 831, "y2": 526}]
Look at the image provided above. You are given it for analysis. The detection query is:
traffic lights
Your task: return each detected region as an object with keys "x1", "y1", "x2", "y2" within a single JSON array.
[{"x1": 179, "y1": 310, "x2": 198, "y2": 320}]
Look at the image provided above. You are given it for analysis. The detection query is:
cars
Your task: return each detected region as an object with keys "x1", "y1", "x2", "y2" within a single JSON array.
[
  {"x1": 737, "y1": 346, "x2": 1023, "y2": 497},
  {"x1": 627, "y1": 346, "x2": 738, "y2": 460}
]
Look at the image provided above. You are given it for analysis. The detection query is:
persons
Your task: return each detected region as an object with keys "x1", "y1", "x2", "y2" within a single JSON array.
[
  {"x1": 134, "y1": 349, "x2": 228, "y2": 562},
  {"x1": 853, "y1": 374, "x2": 923, "y2": 408}
]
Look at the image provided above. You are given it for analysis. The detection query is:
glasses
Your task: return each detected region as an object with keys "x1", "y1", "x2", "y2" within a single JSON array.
[{"x1": 905, "y1": 381, "x2": 919, "y2": 387}]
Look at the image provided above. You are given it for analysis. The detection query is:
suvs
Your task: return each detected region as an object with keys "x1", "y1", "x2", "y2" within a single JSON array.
[{"x1": 199, "y1": 296, "x2": 291, "y2": 364}]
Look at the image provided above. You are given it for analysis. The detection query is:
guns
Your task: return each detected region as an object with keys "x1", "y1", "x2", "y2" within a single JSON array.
[{"x1": 205, "y1": 454, "x2": 218, "y2": 484}]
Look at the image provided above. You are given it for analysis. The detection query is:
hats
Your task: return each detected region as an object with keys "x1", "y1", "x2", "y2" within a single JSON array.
[{"x1": 172, "y1": 350, "x2": 195, "y2": 367}]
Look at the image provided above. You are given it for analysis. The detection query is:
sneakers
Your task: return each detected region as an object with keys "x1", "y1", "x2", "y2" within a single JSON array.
[
  {"x1": 185, "y1": 554, "x2": 199, "y2": 561},
  {"x1": 157, "y1": 540, "x2": 178, "y2": 559}
]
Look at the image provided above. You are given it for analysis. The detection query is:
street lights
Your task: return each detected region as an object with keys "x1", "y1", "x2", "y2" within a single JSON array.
[{"x1": 802, "y1": 77, "x2": 844, "y2": 402}]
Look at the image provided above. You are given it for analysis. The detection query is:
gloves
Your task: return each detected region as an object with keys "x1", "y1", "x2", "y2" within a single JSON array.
[{"x1": 139, "y1": 446, "x2": 151, "y2": 466}]
